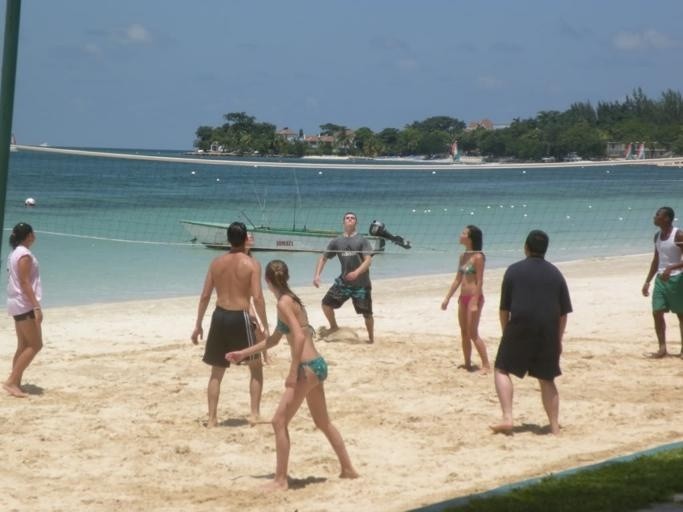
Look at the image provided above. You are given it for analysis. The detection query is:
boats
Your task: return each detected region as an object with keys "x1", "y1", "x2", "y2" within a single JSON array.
[{"x1": 178, "y1": 219, "x2": 409, "y2": 253}]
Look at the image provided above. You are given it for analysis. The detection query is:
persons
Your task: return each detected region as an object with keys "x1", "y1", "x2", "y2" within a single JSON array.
[
  {"x1": 242, "y1": 231, "x2": 272, "y2": 364},
  {"x1": 438, "y1": 224, "x2": 492, "y2": 376},
  {"x1": 225, "y1": 259, "x2": 358, "y2": 492},
  {"x1": 191, "y1": 219, "x2": 271, "y2": 429},
  {"x1": 1, "y1": 222, "x2": 43, "y2": 398},
  {"x1": 313, "y1": 213, "x2": 375, "y2": 343},
  {"x1": 639, "y1": 205, "x2": 683, "y2": 359},
  {"x1": 488, "y1": 230, "x2": 572, "y2": 438}
]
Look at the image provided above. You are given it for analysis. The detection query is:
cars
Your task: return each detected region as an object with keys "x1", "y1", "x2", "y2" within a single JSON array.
[{"x1": 540, "y1": 151, "x2": 582, "y2": 163}]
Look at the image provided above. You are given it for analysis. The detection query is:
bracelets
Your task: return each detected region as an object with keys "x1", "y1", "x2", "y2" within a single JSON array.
[{"x1": 32, "y1": 305, "x2": 41, "y2": 312}]
